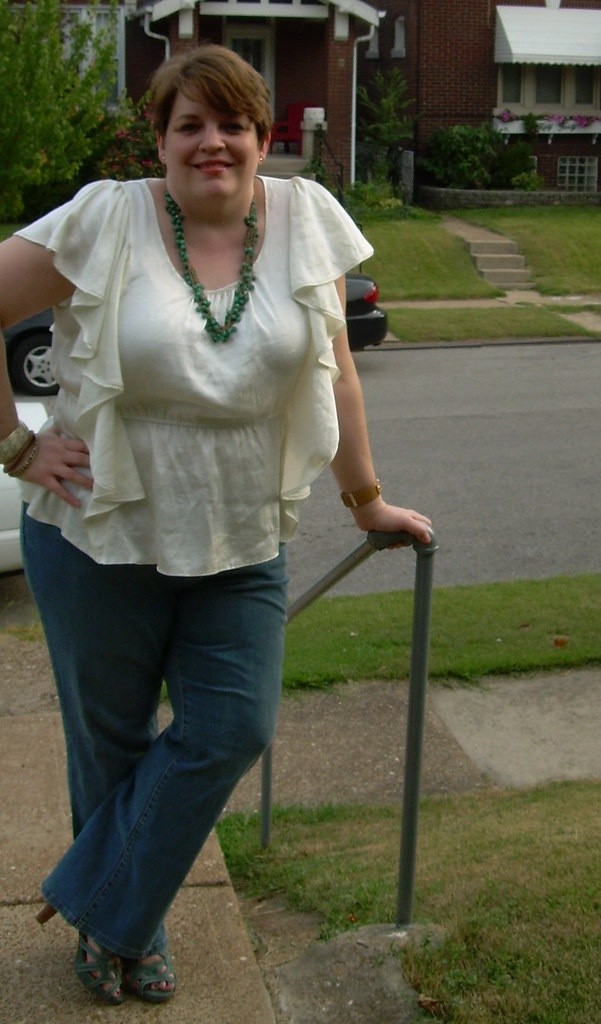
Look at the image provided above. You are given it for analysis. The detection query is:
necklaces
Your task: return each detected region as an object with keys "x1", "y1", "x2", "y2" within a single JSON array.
[{"x1": 162, "y1": 187, "x2": 259, "y2": 340}]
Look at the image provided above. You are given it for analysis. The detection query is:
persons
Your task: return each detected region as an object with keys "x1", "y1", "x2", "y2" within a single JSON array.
[{"x1": 0, "y1": 48, "x2": 433, "y2": 1006}]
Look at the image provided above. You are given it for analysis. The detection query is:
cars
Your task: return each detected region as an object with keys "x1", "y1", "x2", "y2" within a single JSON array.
[{"x1": 2, "y1": 273, "x2": 390, "y2": 399}]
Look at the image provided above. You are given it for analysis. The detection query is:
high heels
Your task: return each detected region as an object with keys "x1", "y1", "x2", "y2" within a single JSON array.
[
  {"x1": 33, "y1": 898, "x2": 127, "y2": 1005},
  {"x1": 122, "y1": 944, "x2": 178, "y2": 1005}
]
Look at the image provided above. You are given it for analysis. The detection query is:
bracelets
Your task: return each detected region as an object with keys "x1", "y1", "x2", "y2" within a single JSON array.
[
  {"x1": 339, "y1": 478, "x2": 382, "y2": 507},
  {"x1": 0, "y1": 419, "x2": 39, "y2": 478}
]
